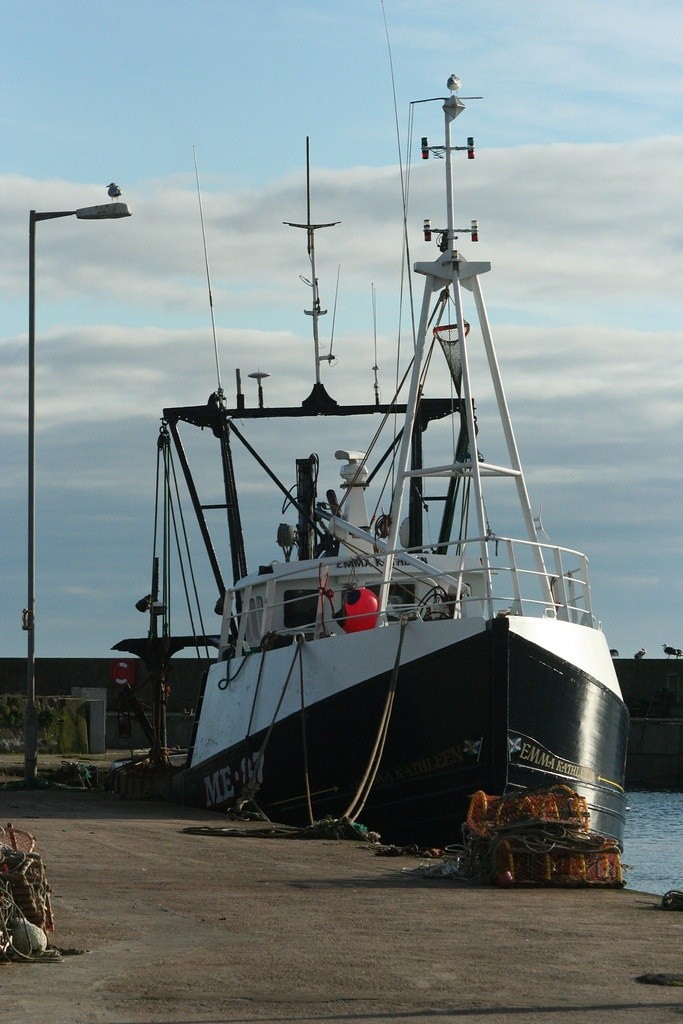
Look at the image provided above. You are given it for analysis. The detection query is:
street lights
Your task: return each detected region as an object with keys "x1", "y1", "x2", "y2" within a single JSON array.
[{"x1": 23, "y1": 201, "x2": 133, "y2": 780}]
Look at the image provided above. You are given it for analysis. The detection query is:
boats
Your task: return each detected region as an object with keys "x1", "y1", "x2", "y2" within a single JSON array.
[{"x1": 190, "y1": 95, "x2": 626, "y2": 862}]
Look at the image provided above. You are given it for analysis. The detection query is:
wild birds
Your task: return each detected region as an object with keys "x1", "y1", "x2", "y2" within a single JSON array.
[
  {"x1": 661, "y1": 643, "x2": 683, "y2": 659},
  {"x1": 634, "y1": 648, "x2": 647, "y2": 659},
  {"x1": 106, "y1": 182, "x2": 123, "y2": 203},
  {"x1": 610, "y1": 649, "x2": 620, "y2": 658}
]
